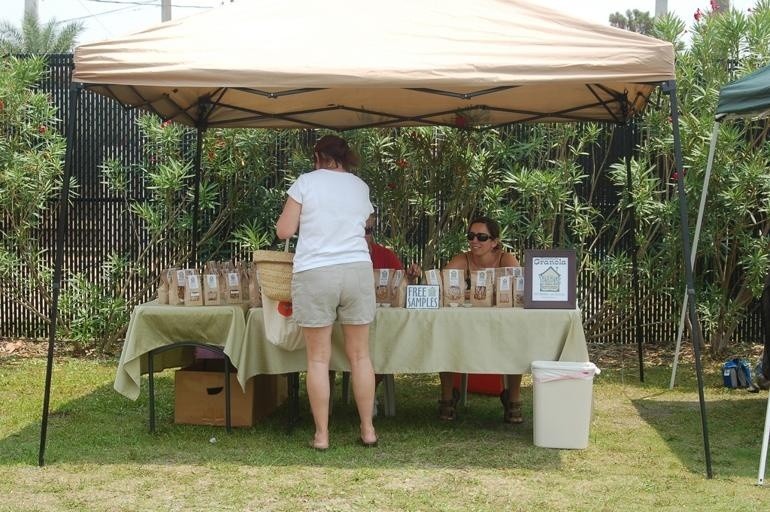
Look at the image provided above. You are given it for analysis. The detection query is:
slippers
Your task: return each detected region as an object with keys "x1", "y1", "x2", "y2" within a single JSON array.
[
  {"x1": 360, "y1": 436, "x2": 379, "y2": 448},
  {"x1": 438, "y1": 387, "x2": 460, "y2": 422},
  {"x1": 310, "y1": 440, "x2": 329, "y2": 451}
]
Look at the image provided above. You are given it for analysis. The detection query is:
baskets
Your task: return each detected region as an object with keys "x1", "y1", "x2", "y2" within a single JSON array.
[{"x1": 253, "y1": 238, "x2": 295, "y2": 302}]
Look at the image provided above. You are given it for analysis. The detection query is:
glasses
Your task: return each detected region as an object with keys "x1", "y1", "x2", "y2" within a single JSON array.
[
  {"x1": 365, "y1": 225, "x2": 373, "y2": 234},
  {"x1": 467, "y1": 232, "x2": 493, "y2": 241}
]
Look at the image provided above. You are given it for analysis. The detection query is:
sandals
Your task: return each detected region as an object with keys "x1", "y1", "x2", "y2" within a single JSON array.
[{"x1": 500, "y1": 389, "x2": 523, "y2": 424}]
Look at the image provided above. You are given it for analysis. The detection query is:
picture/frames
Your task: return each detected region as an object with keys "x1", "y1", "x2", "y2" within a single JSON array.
[{"x1": 524, "y1": 249, "x2": 576, "y2": 309}]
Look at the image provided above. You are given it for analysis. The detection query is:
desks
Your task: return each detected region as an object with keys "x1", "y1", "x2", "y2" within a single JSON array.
[
  {"x1": 114, "y1": 296, "x2": 252, "y2": 433},
  {"x1": 236, "y1": 300, "x2": 590, "y2": 431}
]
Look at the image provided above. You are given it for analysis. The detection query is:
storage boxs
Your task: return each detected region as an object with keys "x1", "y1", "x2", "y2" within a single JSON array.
[{"x1": 173, "y1": 358, "x2": 290, "y2": 428}]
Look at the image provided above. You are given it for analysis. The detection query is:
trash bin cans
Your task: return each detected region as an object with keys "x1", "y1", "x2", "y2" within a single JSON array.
[{"x1": 531, "y1": 360, "x2": 595, "y2": 450}]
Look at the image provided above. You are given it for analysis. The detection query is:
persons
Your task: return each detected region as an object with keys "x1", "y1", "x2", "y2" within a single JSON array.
[
  {"x1": 365, "y1": 215, "x2": 421, "y2": 418},
  {"x1": 436, "y1": 214, "x2": 526, "y2": 427},
  {"x1": 275, "y1": 134, "x2": 379, "y2": 450}
]
